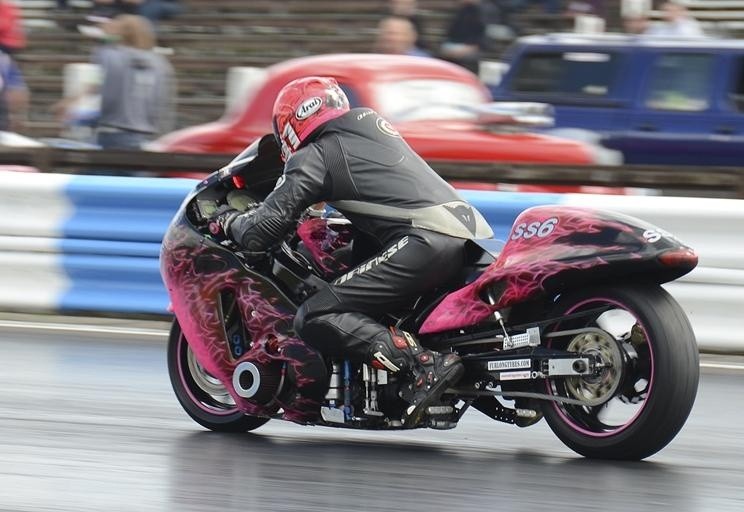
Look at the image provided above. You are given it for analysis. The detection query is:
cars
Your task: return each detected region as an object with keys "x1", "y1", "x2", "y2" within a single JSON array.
[{"x1": 147, "y1": 51, "x2": 630, "y2": 200}]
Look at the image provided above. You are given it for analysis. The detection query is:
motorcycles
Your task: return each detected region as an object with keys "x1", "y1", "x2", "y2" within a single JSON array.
[{"x1": 156, "y1": 129, "x2": 701, "y2": 464}]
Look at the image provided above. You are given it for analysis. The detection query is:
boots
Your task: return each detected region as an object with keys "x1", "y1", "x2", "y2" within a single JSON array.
[{"x1": 367, "y1": 326, "x2": 464, "y2": 428}]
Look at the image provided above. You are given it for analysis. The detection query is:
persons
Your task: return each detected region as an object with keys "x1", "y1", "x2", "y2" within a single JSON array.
[
  {"x1": 569, "y1": 0, "x2": 704, "y2": 39},
  {"x1": 213, "y1": 76, "x2": 494, "y2": 427},
  {"x1": 374, "y1": 0, "x2": 484, "y2": 75},
  {"x1": 52, "y1": 14, "x2": 174, "y2": 150},
  {"x1": 0, "y1": 1, "x2": 28, "y2": 133}
]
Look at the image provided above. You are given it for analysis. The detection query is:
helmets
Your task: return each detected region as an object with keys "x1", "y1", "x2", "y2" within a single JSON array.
[{"x1": 271, "y1": 76, "x2": 351, "y2": 164}]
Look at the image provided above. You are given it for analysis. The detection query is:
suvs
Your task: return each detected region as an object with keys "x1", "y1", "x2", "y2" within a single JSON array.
[{"x1": 483, "y1": 30, "x2": 743, "y2": 198}]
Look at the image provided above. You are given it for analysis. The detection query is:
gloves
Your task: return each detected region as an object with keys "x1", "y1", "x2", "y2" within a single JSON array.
[{"x1": 209, "y1": 204, "x2": 245, "y2": 241}]
